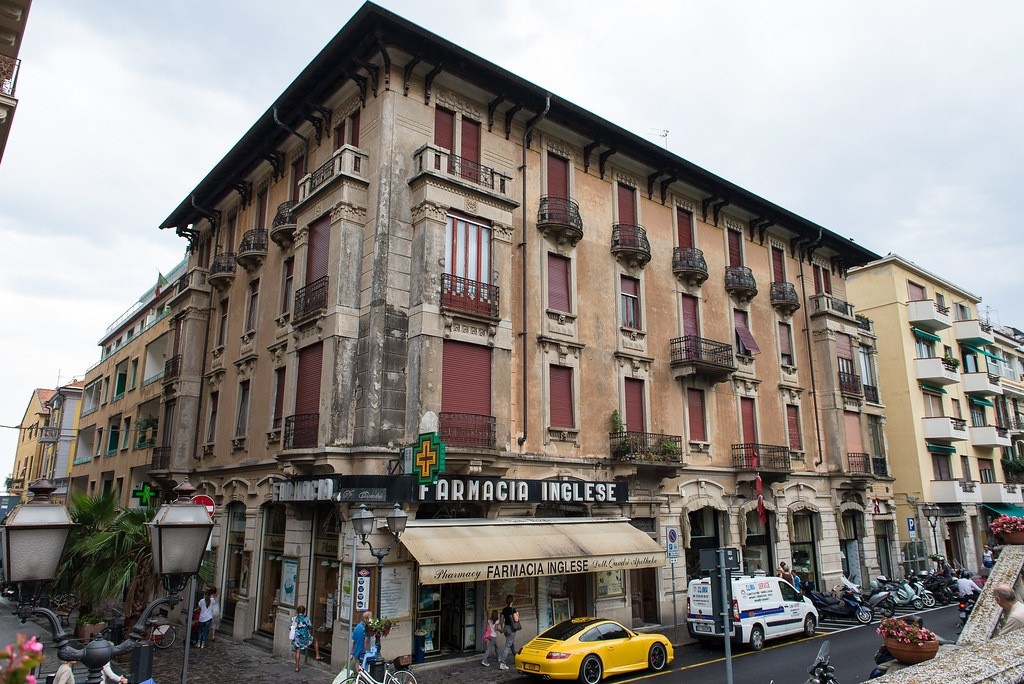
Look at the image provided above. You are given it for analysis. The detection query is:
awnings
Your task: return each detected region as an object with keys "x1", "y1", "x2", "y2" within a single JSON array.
[
  {"x1": 385, "y1": 517, "x2": 666, "y2": 584},
  {"x1": 929, "y1": 444, "x2": 956, "y2": 453},
  {"x1": 970, "y1": 395, "x2": 993, "y2": 407},
  {"x1": 923, "y1": 385, "x2": 947, "y2": 394},
  {"x1": 984, "y1": 504, "x2": 1024, "y2": 518},
  {"x1": 964, "y1": 344, "x2": 1008, "y2": 363},
  {"x1": 913, "y1": 327, "x2": 941, "y2": 342}
]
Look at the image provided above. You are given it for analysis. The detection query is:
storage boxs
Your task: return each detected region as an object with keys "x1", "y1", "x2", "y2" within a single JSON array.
[{"x1": 392, "y1": 654, "x2": 413, "y2": 671}]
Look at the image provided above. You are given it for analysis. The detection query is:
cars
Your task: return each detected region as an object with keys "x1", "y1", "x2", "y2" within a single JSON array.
[{"x1": 514, "y1": 617, "x2": 674, "y2": 684}]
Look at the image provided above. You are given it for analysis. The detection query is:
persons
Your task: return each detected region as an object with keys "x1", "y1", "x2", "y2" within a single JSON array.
[
  {"x1": 956, "y1": 571, "x2": 982, "y2": 597},
  {"x1": 191, "y1": 586, "x2": 220, "y2": 648},
  {"x1": 351, "y1": 611, "x2": 372, "y2": 672},
  {"x1": 53, "y1": 660, "x2": 76, "y2": 684},
  {"x1": 500, "y1": 595, "x2": 520, "y2": 669},
  {"x1": 981, "y1": 544, "x2": 994, "y2": 568},
  {"x1": 753, "y1": 563, "x2": 766, "y2": 575},
  {"x1": 776, "y1": 562, "x2": 795, "y2": 595},
  {"x1": 791, "y1": 570, "x2": 802, "y2": 593},
  {"x1": 993, "y1": 584, "x2": 1024, "y2": 635},
  {"x1": 289, "y1": 606, "x2": 323, "y2": 672},
  {"x1": 481, "y1": 610, "x2": 502, "y2": 667},
  {"x1": 935, "y1": 557, "x2": 951, "y2": 578},
  {"x1": 101, "y1": 662, "x2": 128, "y2": 684}
]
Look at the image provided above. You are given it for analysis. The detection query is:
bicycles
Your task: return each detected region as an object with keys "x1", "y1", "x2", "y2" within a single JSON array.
[
  {"x1": 146, "y1": 619, "x2": 176, "y2": 651},
  {"x1": 340, "y1": 643, "x2": 417, "y2": 684}
]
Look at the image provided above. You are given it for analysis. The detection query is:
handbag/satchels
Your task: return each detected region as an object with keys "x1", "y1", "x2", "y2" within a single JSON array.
[
  {"x1": 510, "y1": 608, "x2": 522, "y2": 631},
  {"x1": 289, "y1": 616, "x2": 297, "y2": 640},
  {"x1": 496, "y1": 624, "x2": 502, "y2": 631}
]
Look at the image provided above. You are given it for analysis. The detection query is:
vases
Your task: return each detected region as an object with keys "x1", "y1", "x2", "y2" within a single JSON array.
[
  {"x1": 611, "y1": 447, "x2": 647, "y2": 460},
  {"x1": 1000, "y1": 531, "x2": 1024, "y2": 544},
  {"x1": 885, "y1": 637, "x2": 940, "y2": 665}
]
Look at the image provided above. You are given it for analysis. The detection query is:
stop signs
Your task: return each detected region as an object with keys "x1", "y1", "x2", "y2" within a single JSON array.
[{"x1": 191, "y1": 495, "x2": 215, "y2": 517}]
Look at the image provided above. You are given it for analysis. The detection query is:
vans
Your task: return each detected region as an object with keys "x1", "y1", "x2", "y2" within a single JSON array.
[{"x1": 685, "y1": 575, "x2": 819, "y2": 651}]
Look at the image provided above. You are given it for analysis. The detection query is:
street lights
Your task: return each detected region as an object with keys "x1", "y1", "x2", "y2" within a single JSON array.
[
  {"x1": 350, "y1": 503, "x2": 409, "y2": 683},
  {"x1": 0, "y1": 474, "x2": 215, "y2": 684},
  {"x1": 921, "y1": 501, "x2": 941, "y2": 571}
]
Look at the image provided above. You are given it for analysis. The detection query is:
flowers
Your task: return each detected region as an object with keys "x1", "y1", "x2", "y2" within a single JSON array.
[
  {"x1": 988, "y1": 512, "x2": 1024, "y2": 537},
  {"x1": 362, "y1": 614, "x2": 394, "y2": 640},
  {"x1": 876, "y1": 615, "x2": 936, "y2": 646},
  {"x1": 609, "y1": 409, "x2": 655, "y2": 466}
]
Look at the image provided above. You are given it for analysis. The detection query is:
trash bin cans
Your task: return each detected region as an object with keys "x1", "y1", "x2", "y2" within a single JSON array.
[
  {"x1": 370, "y1": 660, "x2": 386, "y2": 684},
  {"x1": 414, "y1": 629, "x2": 427, "y2": 662}
]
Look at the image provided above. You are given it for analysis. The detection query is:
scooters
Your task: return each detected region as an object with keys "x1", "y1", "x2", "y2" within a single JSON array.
[
  {"x1": 811, "y1": 559, "x2": 984, "y2": 634},
  {"x1": 770, "y1": 640, "x2": 839, "y2": 684}
]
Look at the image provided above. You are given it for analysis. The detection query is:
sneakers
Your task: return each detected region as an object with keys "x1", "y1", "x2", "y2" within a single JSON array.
[{"x1": 500, "y1": 663, "x2": 510, "y2": 670}]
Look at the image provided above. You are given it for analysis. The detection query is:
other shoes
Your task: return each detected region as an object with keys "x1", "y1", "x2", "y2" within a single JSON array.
[
  {"x1": 497, "y1": 658, "x2": 502, "y2": 662},
  {"x1": 196, "y1": 642, "x2": 206, "y2": 649},
  {"x1": 295, "y1": 668, "x2": 300, "y2": 672},
  {"x1": 481, "y1": 660, "x2": 490, "y2": 667},
  {"x1": 316, "y1": 656, "x2": 324, "y2": 661}
]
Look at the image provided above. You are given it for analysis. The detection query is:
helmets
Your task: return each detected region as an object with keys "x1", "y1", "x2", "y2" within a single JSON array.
[
  {"x1": 961, "y1": 570, "x2": 969, "y2": 579},
  {"x1": 984, "y1": 545, "x2": 989, "y2": 548},
  {"x1": 938, "y1": 557, "x2": 945, "y2": 561}
]
